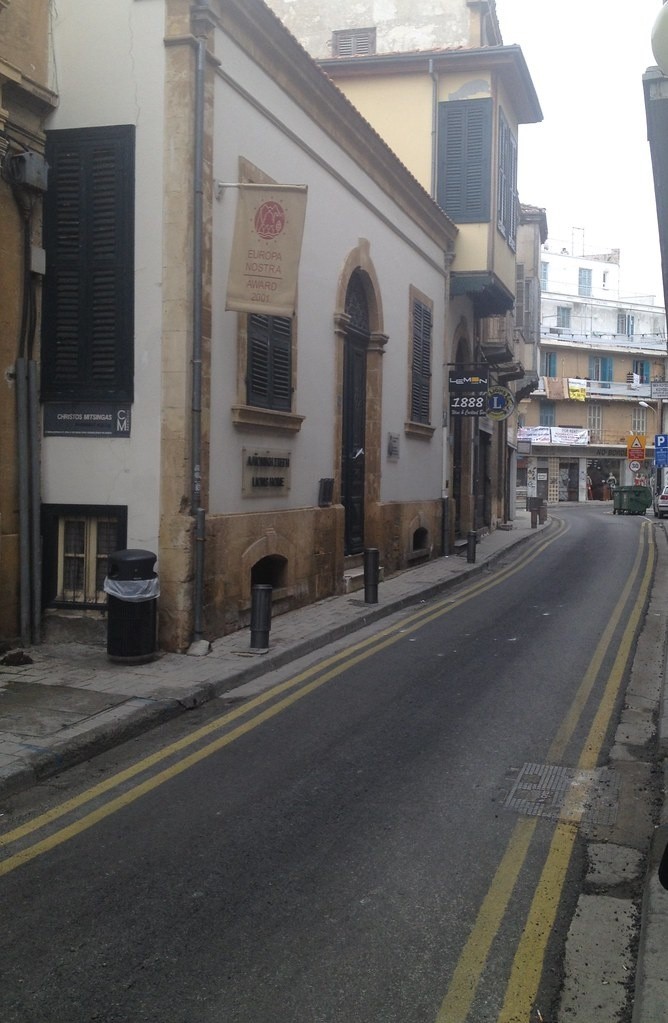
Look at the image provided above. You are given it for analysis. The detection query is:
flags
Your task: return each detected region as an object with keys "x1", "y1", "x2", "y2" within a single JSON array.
[{"x1": 225, "y1": 183, "x2": 308, "y2": 320}]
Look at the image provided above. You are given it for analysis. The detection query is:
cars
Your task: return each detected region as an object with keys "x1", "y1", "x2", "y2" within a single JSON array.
[{"x1": 653, "y1": 485, "x2": 668, "y2": 519}]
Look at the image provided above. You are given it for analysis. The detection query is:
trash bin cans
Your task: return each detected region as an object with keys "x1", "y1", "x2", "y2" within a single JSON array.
[
  {"x1": 104, "y1": 548, "x2": 161, "y2": 665},
  {"x1": 612, "y1": 486, "x2": 653, "y2": 515}
]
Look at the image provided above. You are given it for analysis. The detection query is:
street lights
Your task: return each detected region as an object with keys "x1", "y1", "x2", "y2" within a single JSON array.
[{"x1": 638, "y1": 400, "x2": 662, "y2": 496}]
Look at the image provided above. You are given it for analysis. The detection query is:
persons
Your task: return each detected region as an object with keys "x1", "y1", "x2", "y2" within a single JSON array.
[
  {"x1": 587, "y1": 473, "x2": 593, "y2": 500},
  {"x1": 607, "y1": 472, "x2": 617, "y2": 500}
]
